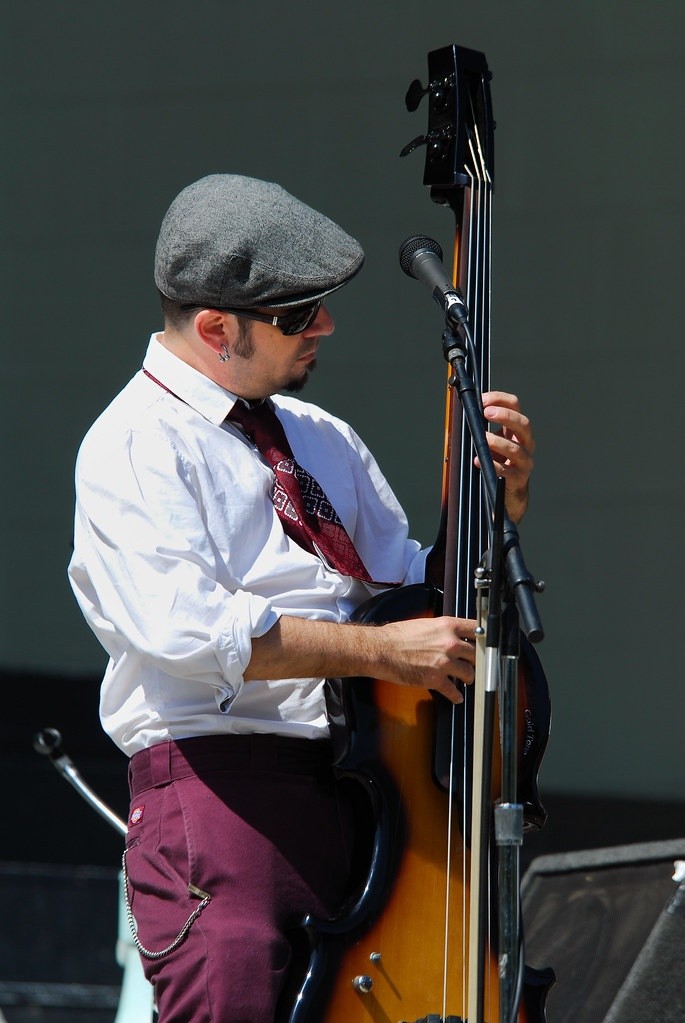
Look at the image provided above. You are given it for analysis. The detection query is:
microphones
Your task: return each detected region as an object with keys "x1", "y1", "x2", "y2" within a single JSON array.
[{"x1": 398, "y1": 232, "x2": 468, "y2": 325}]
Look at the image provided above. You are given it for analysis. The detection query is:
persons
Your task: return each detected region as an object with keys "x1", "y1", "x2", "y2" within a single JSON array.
[{"x1": 68, "y1": 177, "x2": 536, "y2": 1023}]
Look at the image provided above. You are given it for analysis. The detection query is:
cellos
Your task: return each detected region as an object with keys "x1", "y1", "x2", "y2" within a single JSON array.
[{"x1": 276, "y1": 45, "x2": 558, "y2": 1023}]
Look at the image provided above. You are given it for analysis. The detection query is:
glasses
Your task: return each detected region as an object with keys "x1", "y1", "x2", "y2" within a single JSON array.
[{"x1": 220, "y1": 296, "x2": 327, "y2": 336}]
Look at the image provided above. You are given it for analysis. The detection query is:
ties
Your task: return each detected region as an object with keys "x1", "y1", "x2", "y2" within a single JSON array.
[{"x1": 229, "y1": 400, "x2": 373, "y2": 584}]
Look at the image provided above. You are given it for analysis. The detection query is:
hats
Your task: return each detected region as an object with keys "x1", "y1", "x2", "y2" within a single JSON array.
[{"x1": 154, "y1": 174, "x2": 366, "y2": 308}]
